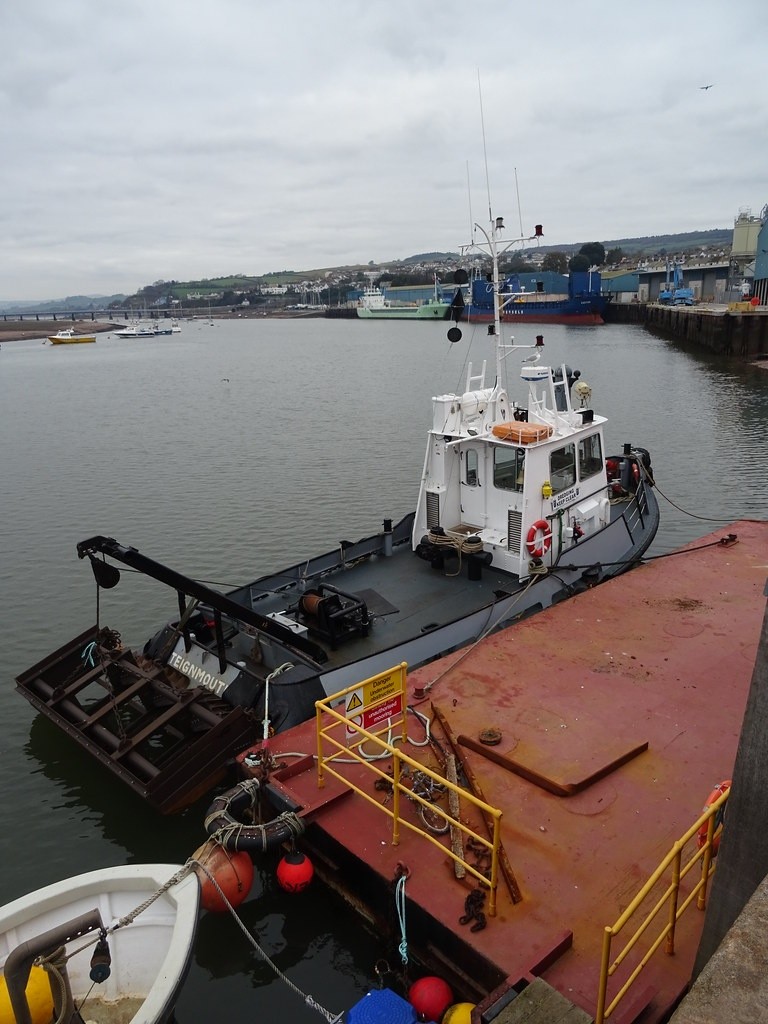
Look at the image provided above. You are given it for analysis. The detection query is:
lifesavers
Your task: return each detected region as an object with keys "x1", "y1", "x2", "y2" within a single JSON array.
[{"x1": 525, "y1": 520, "x2": 553, "y2": 558}]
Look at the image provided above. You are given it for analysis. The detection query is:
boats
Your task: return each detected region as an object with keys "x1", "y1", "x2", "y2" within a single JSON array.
[
  {"x1": 47, "y1": 327, "x2": 95, "y2": 344},
  {"x1": 460, "y1": 265, "x2": 614, "y2": 325},
  {"x1": 204, "y1": 519, "x2": 768, "y2": 1024},
  {"x1": 14, "y1": 71, "x2": 659, "y2": 813},
  {"x1": 0, "y1": 864, "x2": 199, "y2": 1024},
  {"x1": 113, "y1": 297, "x2": 181, "y2": 338},
  {"x1": 356, "y1": 273, "x2": 450, "y2": 318}
]
